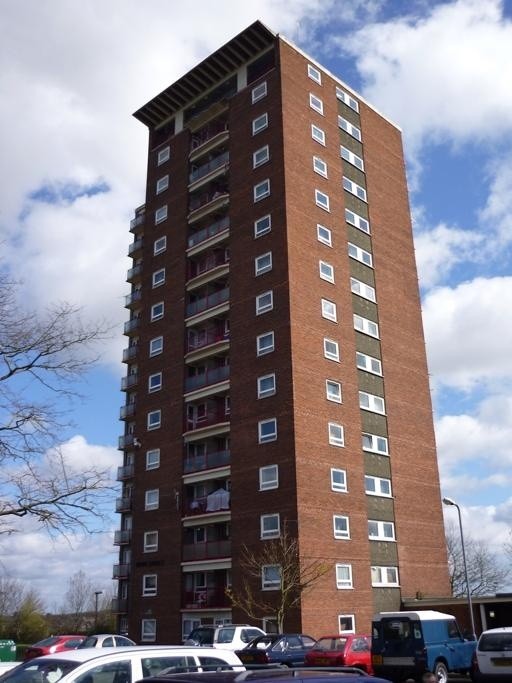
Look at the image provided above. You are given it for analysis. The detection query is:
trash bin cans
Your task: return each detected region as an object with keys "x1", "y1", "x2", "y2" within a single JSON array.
[{"x1": 0, "y1": 639, "x2": 17, "y2": 662}]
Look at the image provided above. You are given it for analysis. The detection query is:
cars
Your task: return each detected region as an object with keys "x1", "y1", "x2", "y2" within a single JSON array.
[
  {"x1": 472, "y1": 626, "x2": 512, "y2": 681},
  {"x1": 0, "y1": 633, "x2": 246, "y2": 683},
  {"x1": 238, "y1": 633, "x2": 321, "y2": 667},
  {"x1": 305, "y1": 633, "x2": 373, "y2": 676}
]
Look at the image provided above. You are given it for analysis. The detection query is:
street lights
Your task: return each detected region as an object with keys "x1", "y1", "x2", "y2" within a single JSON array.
[
  {"x1": 94, "y1": 590, "x2": 102, "y2": 629},
  {"x1": 443, "y1": 498, "x2": 477, "y2": 639}
]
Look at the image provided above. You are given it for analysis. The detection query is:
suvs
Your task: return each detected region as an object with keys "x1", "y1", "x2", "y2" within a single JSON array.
[
  {"x1": 370, "y1": 611, "x2": 478, "y2": 682},
  {"x1": 134, "y1": 662, "x2": 391, "y2": 683},
  {"x1": 185, "y1": 623, "x2": 288, "y2": 653}
]
